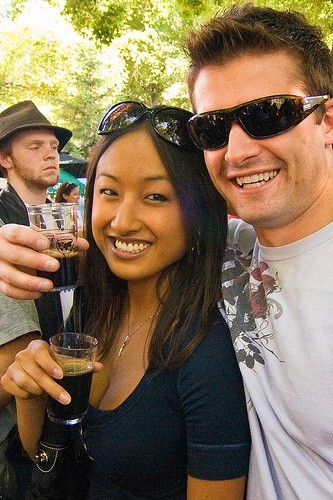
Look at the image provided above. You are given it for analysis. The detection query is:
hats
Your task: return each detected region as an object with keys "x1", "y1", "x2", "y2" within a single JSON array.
[{"x1": 0, "y1": 100, "x2": 72, "y2": 178}]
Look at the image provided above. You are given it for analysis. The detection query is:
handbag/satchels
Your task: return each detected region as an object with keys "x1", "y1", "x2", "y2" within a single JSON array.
[{"x1": 24, "y1": 422, "x2": 91, "y2": 500}]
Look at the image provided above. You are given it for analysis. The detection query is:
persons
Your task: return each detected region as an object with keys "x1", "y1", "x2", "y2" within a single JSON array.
[
  {"x1": 1, "y1": 7, "x2": 333, "y2": 499},
  {"x1": 0, "y1": 99, "x2": 98, "y2": 500},
  {"x1": 0, "y1": 96, "x2": 252, "y2": 500}
]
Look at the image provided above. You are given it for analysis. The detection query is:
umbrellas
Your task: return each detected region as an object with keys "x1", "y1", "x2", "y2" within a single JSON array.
[
  {"x1": 57, "y1": 151, "x2": 88, "y2": 179},
  {"x1": 47, "y1": 167, "x2": 87, "y2": 196}
]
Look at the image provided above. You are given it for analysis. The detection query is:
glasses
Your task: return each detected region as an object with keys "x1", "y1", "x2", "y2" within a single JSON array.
[
  {"x1": 65, "y1": 181, "x2": 74, "y2": 188},
  {"x1": 96, "y1": 100, "x2": 197, "y2": 152},
  {"x1": 187, "y1": 94, "x2": 330, "y2": 151}
]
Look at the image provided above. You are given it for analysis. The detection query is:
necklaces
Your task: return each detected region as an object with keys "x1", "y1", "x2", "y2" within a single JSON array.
[{"x1": 119, "y1": 304, "x2": 161, "y2": 355}]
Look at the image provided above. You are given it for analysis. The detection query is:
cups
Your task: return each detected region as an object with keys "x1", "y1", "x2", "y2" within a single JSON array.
[
  {"x1": 47, "y1": 332, "x2": 98, "y2": 425},
  {"x1": 25, "y1": 201, "x2": 78, "y2": 290}
]
